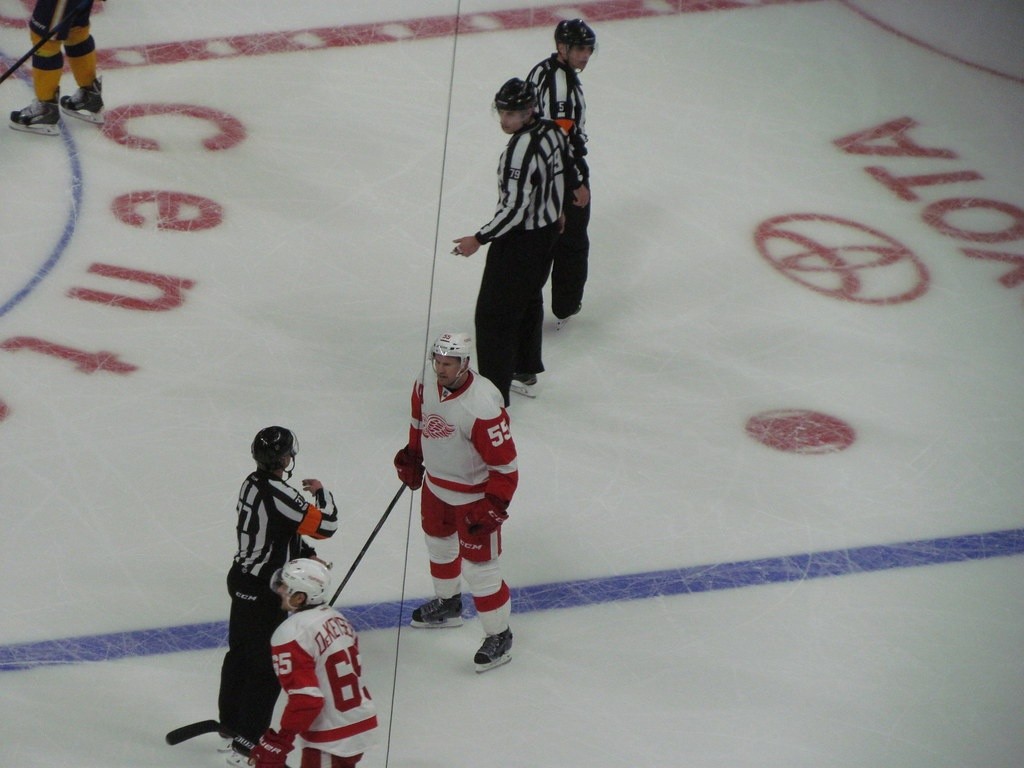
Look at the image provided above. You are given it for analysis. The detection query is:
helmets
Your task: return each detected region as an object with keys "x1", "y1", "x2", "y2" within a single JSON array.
[
  {"x1": 494, "y1": 78, "x2": 539, "y2": 111},
  {"x1": 428, "y1": 332, "x2": 474, "y2": 358},
  {"x1": 251, "y1": 425, "x2": 294, "y2": 471},
  {"x1": 280, "y1": 558, "x2": 331, "y2": 606},
  {"x1": 554, "y1": 18, "x2": 595, "y2": 47}
]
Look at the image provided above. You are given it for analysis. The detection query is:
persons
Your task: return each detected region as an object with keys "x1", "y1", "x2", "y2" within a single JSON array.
[
  {"x1": 247, "y1": 558, "x2": 378, "y2": 768},
  {"x1": 218, "y1": 426, "x2": 339, "y2": 768},
  {"x1": 394, "y1": 332, "x2": 520, "y2": 672},
  {"x1": 9, "y1": 0, "x2": 105, "y2": 135},
  {"x1": 451, "y1": 77, "x2": 569, "y2": 406},
  {"x1": 526, "y1": 18, "x2": 595, "y2": 331}
]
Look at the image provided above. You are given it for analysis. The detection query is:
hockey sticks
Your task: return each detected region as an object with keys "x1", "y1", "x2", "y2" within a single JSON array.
[
  {"x1": 328, "y1": 482, "x2": 407, "y2": 607},
  {"x1": 166, "y1": 720, "x2": 292, "y2": 768},
  {"x1": 0, "y1": 0, "x2": 93, "y2": 84}
]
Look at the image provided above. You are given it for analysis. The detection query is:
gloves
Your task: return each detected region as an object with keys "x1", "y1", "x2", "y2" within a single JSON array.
[
  {"x1": 248, "y1": 730, "x2": 295, "y2": 767},
  {"x1": 393, "y1": 446, "x2": 424, "y2": 491},
  {"x1": 464, "y1": 497, "x2": 509, "y2": 538}
]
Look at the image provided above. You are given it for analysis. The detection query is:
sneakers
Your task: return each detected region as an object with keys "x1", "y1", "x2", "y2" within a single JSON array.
[
  {"x1": 10, "y1": 86, "x2": 60, "y2": 125},
  {"x1": 60, "y1": 77, "x2": 104, "y2": 113},
  {"x1": 511, "y1": 372, "x2": 537, "y2": 385},
  {"x1": 474, "y1": 625, "x2": 513, "y2": 664},
  {"x1": 232, "y1": 728, "x2": 267, "y2": 758},
  {"x1": 553, "y1": 303, "x2": 582, "y2": 319},
  {"x1": 412, "y1": 592, "x2": 463, "y2": 623}
]
